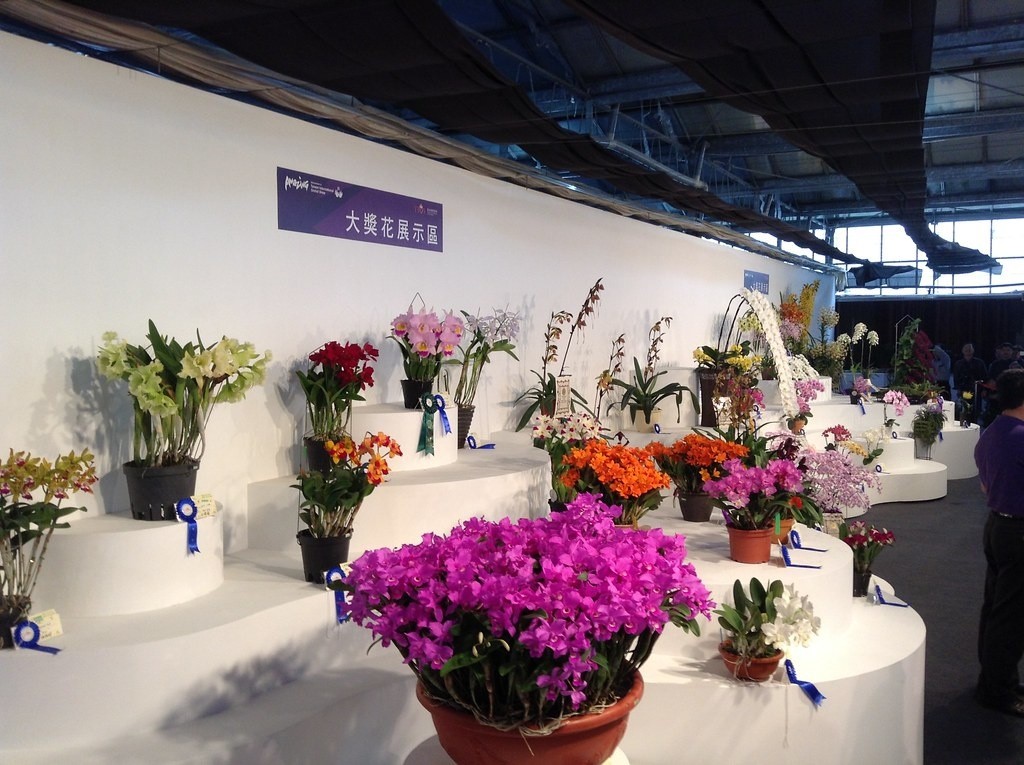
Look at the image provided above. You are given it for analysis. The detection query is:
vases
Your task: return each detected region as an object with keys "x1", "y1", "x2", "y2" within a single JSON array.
[
  {"x1": 849, "y1": 390, "x2": 860, "y2": 405},
  {"x1": 853, "y1": 571, "x2": 873, "y2": 597},
  {"x1": 548, "y1": 498, "x2": 568, "y2": 518},
  {"x1": 0, "y1": 594, "x2": 31, "y2": 648},
  {"x1": 696, "y1": 368, "x2": 719, "y2": 427},
  {"x1": 413, "y1": 659, "x2": 643, "y2": 765},
  {"x1": 118, "y1": 457, "x2": 201, "y2": 522},
  {"x1": 819, "y1": 511, "x2": 845, "y2": 538},
  {"x1": 454, "y1": 404, "x2": 476, "y2": 449},
  {"x1": 633, "y1": 409, "x2": 661, "y2": 433},
  {"x1": 718, "y1": 636, "x2": 784, "y2": 682},
  {"x1": 400, "y1": 378, "x2": 434, "y2": 409},
  {"x1": 677, "y1": 492, "x2": 716, "y2": 522},
  {"x1": 791, "y1": 420, "x2": 805, "y2": 434},
  {"x1": 724, "y1": 523, "x2": 775, "y2": 564},
  {"x1": 295, "y1": 526, "x2": 355, "y2": 584},
  {"x1": 761, "y1": 368, "x2": 775, "y2": 381},
  {"x1": 302, "y1": 435, "x2": 349, "y2": 479},
  {"x1": 768, "y1": 518, "x2": 795, "y2": 544},
  {"x1": 613, "y1": 524, "x2": 634, "y2": 531}
]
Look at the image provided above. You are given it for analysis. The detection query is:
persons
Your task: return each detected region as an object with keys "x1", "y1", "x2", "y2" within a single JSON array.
[
  {"x1": 953, "y1": 344, "x2": 988, "y2": 424},
  {"x1": 974, "y1": 367, "x2": 1024, "y2": 720},
  {"x1": 930, "y1": 341, "x2": 951, "y2": 402},
  {"x1": 986, "y1": 342, "x2": 1024, "y2": 377}
]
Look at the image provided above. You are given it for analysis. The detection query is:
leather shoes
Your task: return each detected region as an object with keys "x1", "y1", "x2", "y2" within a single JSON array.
[
  {"x1": 1016, "y1": 683, "x2": 1024, "y2": 695},
  {"x1": 986, "y1": 696, "x2": 1024, "y2": 717}
]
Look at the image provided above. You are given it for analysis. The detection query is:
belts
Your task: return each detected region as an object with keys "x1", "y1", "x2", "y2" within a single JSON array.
[{"x1": 997, "y1": 513, "x2": 1013, "y2": 518}]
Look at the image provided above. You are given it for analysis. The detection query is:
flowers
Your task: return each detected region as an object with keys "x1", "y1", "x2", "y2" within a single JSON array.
[
  {"x1": 842, "y1": 520, "x2": 897, "y2": 572},
  {"x1": 513, "y1": 276, "x2": 973, "y2": 530},
  {"x1": 436, "y1": 303, "x2": 524, "y2": 405},
  {"x1": 712, "y1": 575, "x2": 823, "y2": 658},
  {"x1": 341, "y1": 493, "x2": 715, "y2": 723},
  {"x1": 96, "y1": 319, "x2": 280, "y2": 465},
  {"x1": 295, "y1": 339, "x2": 380, "y2": 436},
  {"x1": 289, "y1": 433, "x2": 404, "y2": 538},
  {"x1": 0, "y1": 447, "x2": 99, "y2": 603},
  {"x1": 384, "y1": 304, "x2": 466, "y2": 380}
]
